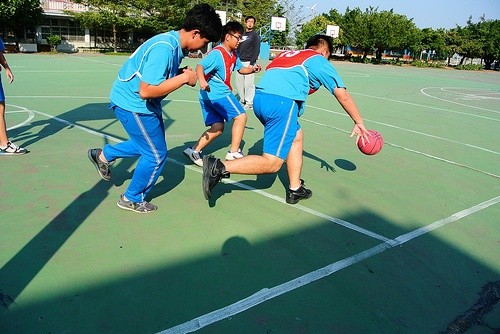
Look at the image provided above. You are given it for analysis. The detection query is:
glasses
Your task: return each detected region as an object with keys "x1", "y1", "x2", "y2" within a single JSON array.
[{"x1": 230, "y1": 33, "x2": 242, "y2": 41}]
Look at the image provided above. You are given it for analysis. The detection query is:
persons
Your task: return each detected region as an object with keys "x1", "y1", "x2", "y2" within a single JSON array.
[
  {"x1": 88, "y1": 3, "x2": 224, "y2": 213},
  {"x1": 202, "y1": 34, "x2": 371, "y2": 204},
  {"x1": 235, "y1": 16, "x2": 261, "y2": 110},
  {"x1": 0, "y1": 37, "x2": 27, "y2": 155},
  {"x1": 183, "y1": 20, "x2": 263, "y2": 167}
]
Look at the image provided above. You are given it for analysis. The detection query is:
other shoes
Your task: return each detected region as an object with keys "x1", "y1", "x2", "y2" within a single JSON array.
[
  {"x1": 243, "y1": 105, "x2": 254, "y2": 111},
  {"x1": 238, "y1": 101, "x2": 244, "y2": 107}
]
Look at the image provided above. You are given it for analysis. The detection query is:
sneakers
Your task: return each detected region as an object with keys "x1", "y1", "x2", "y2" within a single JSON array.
[
  {"x1": 224, "y1": 148, "x2": 244, "y2": 161},
  {"x1": 285, "y1": 178, "x2": 313, "y2": 205},
  {"x1": 182, "y1": 146, "x2": 204, "y2": 167},
  {"x1": 117, "y1": 194, "x2": 157, "y2": 215},
  {"x1": 0, "y1": 140, "x2": 28, "y2": 156},
  {"x1": 87, "y1": 148, "x2": 113, "y2": 182},
  {"x1": 202, "y1": 155, "x2": 230, "y2": 201}
]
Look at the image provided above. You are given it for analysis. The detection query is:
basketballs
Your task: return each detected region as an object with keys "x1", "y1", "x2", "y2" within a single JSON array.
[{"x1": 358, "y1": 130, "x2": 383, "y2": 155}]
